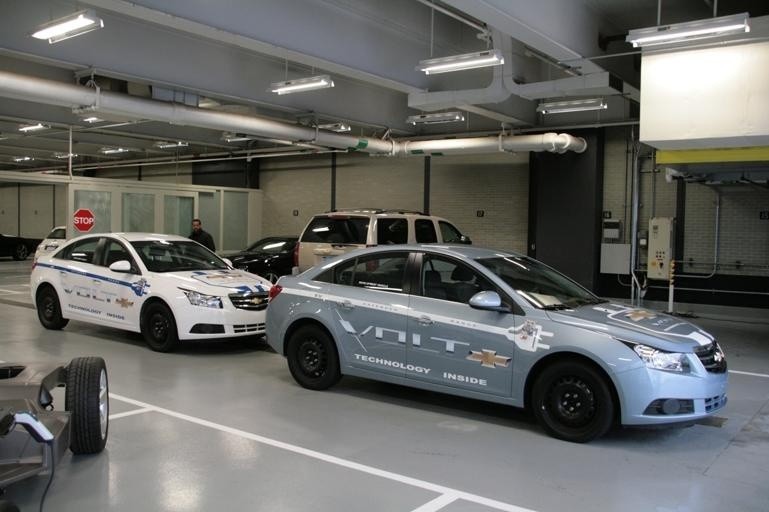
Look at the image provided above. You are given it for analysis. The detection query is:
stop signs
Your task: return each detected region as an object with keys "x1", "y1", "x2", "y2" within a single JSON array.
[{"x1": 73, "y1": 208, "x2": 96, "y2": 232}]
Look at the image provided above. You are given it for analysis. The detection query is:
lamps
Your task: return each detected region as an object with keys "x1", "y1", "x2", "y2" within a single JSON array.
[
  {"x1": 624, "y1": 1, "x2": 751, "y2": 50},
  {"x1": 415, "y1": 1, "x2": 505, "y2": 76},
  {"x1": 535, "y1": 63, "x2": 608, "y2": 115},
  {"x1": 405, "y1": 108, "x2": 465, "y2": 127},
  {"x1": 25, "y1": 0, "x2": 104, "y2": 47},
  {"x1": 270, "y1": 48, "x2": 334, "y2": 96},
  {"x1": 315, "y1": 122, "x2": 351, "y2": 134}
]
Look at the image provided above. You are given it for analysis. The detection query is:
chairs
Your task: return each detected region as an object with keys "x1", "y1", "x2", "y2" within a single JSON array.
[
  {"x1": 424, "y1": 270, "x2": 449, "y2": 299},
  {"x1": 450, "y1": 266, "x2": 479, "y2": 303},
  {"x1": 148, "y1": 246, "x2": 170, "y2": 271}
]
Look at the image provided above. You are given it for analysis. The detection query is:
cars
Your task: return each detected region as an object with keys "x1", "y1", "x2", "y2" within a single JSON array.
[
  {"x1": 220, "y1": 235, "x2": 298, "y2": 282},
  {"x1": 262, "y1": 240, "x2": 730, "y2": 443},
  {"x1": 30, "y1": 232, "x2": 277, "y2": 352},
  {"x1": 34, "y1": 226, "x2": 66, "y2": 268},
  {"x1": 0, "y1": 234, "x2": 33, "y2": 261}
]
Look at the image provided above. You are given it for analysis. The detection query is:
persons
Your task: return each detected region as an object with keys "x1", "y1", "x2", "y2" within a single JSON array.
[{"x1": 182, "y1": 218, "x2": 217, "y2": 270}]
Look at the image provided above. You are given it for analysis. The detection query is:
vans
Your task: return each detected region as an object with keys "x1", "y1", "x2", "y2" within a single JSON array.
[{"x1": 292, "y1": 207, "x2": 470, "y2": 286}]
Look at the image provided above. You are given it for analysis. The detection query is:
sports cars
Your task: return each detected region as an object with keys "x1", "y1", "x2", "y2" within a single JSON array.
[{"x1": 0, "y1": 355, "x2": 111, "y2": 511}]
